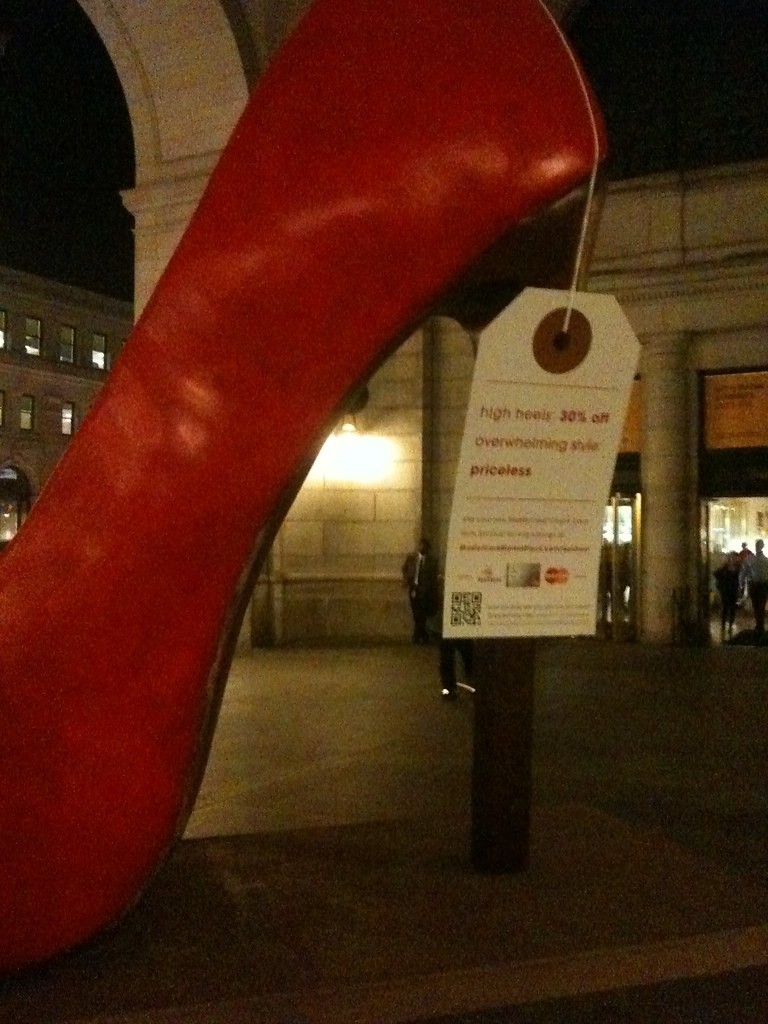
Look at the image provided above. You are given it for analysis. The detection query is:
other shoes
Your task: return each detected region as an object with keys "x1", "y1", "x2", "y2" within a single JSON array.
[{"x1": 412, "y1": 633, "x2": 428, "y2": 645}]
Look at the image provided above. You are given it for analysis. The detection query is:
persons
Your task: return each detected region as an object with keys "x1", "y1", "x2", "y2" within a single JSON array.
[
  {"x1": 714, "y1": 539, "x2": 768, "y2": 645},
  {"x1": 402, "y1": 538, "x2": 440, "y2": 644},
  {"x1": 439, "y1": 575, "x2": 477, "y2": 697}
]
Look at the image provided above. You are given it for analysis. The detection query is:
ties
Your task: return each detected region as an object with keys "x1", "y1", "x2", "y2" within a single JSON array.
[{"x1": 418, "y1": 557, "x2": 423, "y2": 583}]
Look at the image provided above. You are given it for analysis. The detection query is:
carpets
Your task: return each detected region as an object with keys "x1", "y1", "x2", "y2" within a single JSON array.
[{"x1": 726, "y1": 627, "x2": 768, "y2": 647}]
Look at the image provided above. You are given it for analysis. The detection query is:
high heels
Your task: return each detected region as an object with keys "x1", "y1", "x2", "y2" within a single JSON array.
[{"x1": 0, "y1": 0, "x2": 611, "y2": 972}]
